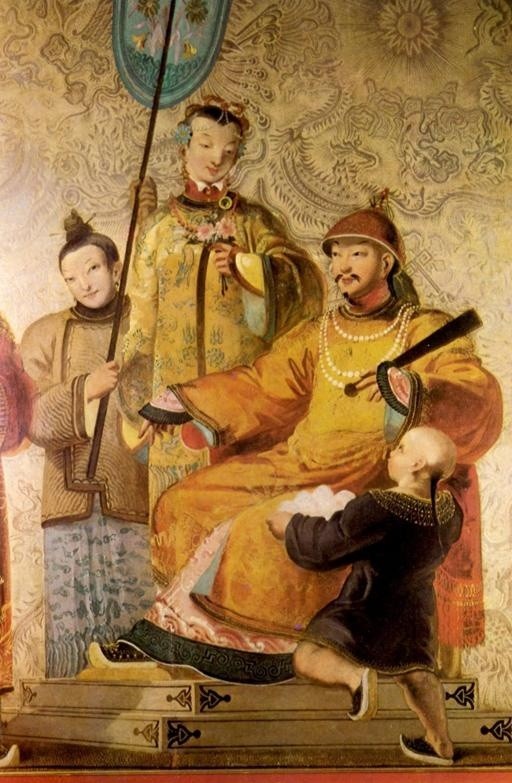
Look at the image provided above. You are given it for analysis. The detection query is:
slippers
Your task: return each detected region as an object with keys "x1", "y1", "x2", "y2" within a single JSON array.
[
  {"x1": 399, "y1": 733, "x2": 455, "y2": 767},
  {"x1": 346, "y1": 666, "x2": 379, "y2": 722}
]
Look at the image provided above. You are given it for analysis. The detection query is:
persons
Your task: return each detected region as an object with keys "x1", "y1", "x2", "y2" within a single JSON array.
[
  {"x1": 16, "y1": 176, "x2": 157, "y2": 678},
  {"x1": 91, "y1": 208, "x2": 503, "y2": 685},
  {"x1": 113, "y1": 105, "x2": 329, "y2": 532},
  {"x1": 267, "y1": 426, "x2": 464, "y2": 767},
  {"x1": 0, "y1": 316, "x2": 40, "y2": 697}
]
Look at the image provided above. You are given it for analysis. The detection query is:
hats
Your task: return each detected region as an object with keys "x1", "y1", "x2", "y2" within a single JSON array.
[{"x1": 319, "y1": 188, "x2": 407, "y2": 276}]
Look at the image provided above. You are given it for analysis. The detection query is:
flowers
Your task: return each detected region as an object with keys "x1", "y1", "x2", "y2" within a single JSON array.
[{"x1": 194, "y1": 214, "x2": 239, "y2": 299}]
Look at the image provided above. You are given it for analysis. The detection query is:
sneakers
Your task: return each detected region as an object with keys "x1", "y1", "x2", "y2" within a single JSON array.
[
  {"x1": 88, "y1": 640, "x2": 158, "y2": 671},
  {"x1": 0, "y1": 744, "x2": 21, "y2": 768}
]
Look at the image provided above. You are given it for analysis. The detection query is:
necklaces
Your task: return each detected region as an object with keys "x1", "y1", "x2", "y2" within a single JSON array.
[
  {"x1": 323, "y1": 301, "x2": 419, "y2": 377},
  {"x1": 172, "y1": 195, "x2": 239, "y2": 235},
  {"x1": 331, "y1": 300, "x2": 413, "y2": 342},
  {"x1": 317, "y1": 310, "x2": 415, "y2": 389}
]
